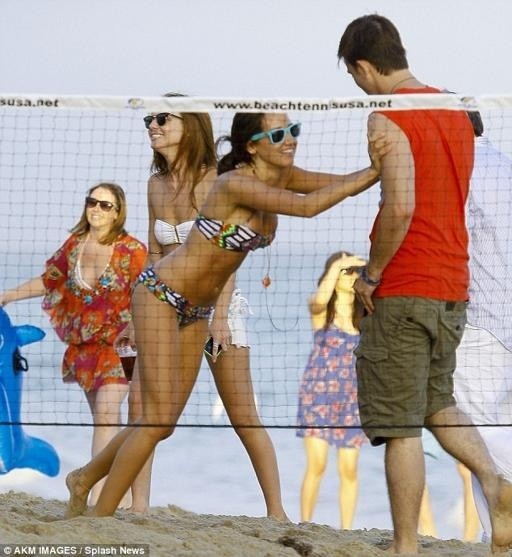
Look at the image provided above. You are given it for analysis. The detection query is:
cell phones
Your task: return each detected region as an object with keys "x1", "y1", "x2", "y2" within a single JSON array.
[{"x1": 202, "y1": 336, "x2": 223, "y2": 359}]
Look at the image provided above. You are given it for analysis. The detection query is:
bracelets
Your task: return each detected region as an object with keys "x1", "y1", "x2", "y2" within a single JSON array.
[
  {"x1": 147, "y1": 251, "x2": 164, "y2": 256},
  {"x1": 359, "y1": 268, "x2": 380, "y2": 285}
]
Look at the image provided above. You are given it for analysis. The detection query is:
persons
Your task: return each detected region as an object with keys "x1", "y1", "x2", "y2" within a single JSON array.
[
  {"x1": 129, "y1": 92, "x2": 292, "y2": 526},
  {"x1": 65, "y1": 111, "x2": 392, "y2": 522},
  {"x1": 339, "y1": 12, "x2": 512, "y2": 556},
  {"x1": 297, "y1": 251, "x2": 368, "y2": 532},
  {"x1": 0, "y1": 182, "x2": 149, "y2": 505}
]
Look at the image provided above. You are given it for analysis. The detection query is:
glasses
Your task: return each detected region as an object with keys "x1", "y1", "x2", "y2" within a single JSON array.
[
  {"x1": 143, "y1": 112, "x2": 183, "y2": 129},
  {"x1": 252, "y1": 121, "x2": 301, "y2": 146},
  {"x1": 341, "y1": 267, "x2": 364, "y2": 276},
  {"x1": 85, "y1": 197, "x2": 119, "y2": 212}
]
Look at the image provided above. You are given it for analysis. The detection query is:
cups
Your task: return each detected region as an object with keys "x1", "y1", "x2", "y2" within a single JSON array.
[{"x1": 117, "y1": 343, "x2": 138, "y2": 382}]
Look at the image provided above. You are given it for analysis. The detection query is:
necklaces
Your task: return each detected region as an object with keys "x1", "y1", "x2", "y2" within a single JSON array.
[{"x1": 391, "y1": 77, "x2": 417, "y2": 92}]
